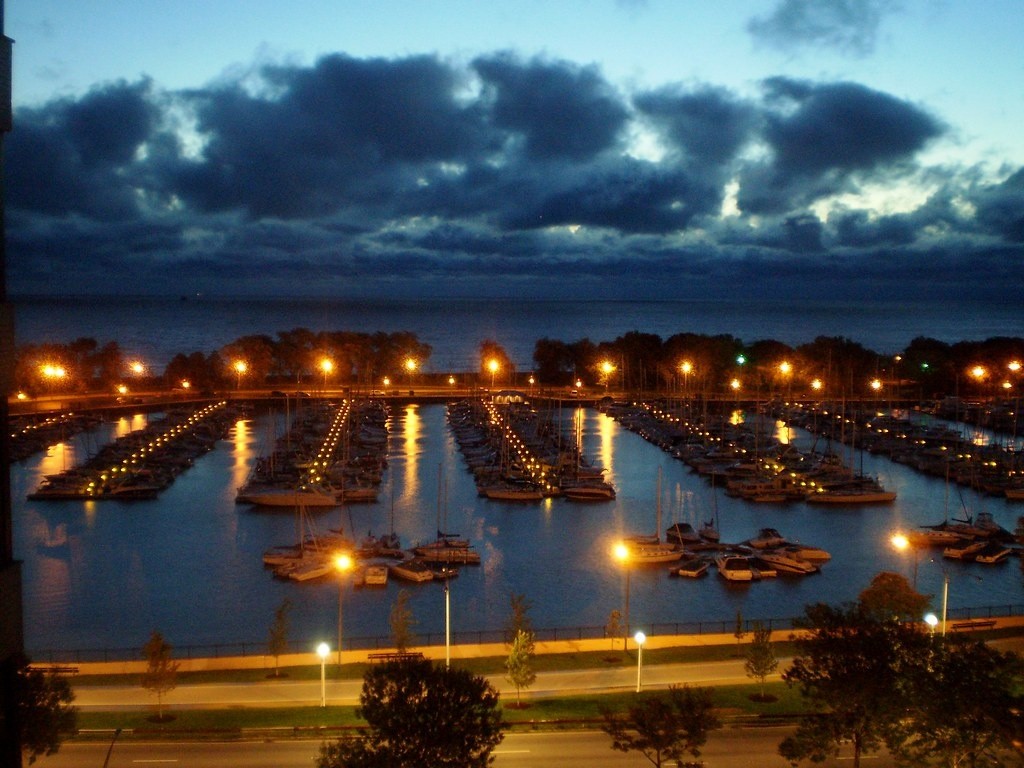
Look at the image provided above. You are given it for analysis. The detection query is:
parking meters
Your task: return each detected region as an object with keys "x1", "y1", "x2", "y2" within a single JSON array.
[{"x1": 574, "y1": 378, "x2": 583, "y2": 400}]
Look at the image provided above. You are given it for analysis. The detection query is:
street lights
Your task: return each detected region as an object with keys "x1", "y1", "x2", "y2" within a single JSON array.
[
  {"x1": 320, "y1": 360, "x2": 334, "y2": 393},
  {"x1": 612, "y1": 540, "x2": 629, "y2": 652},
  {"x1": 919, "y1": 363, "x2": 929, "y2": 400},
  {"x1": 529, "y1": 375, "x2": 534, "y2": 397},
  {"x1": 42, "y1": 363, "x2": 69, "y2": 396},
  {"x1": 182, "y1": 380, "x2": 190, "y2": 391},
  {"x1": 736, "y1": 354, "x2": 747, "y2": 378},
  {"x1": 811, "y1": 379, "x2": 824, "y2": 401},
  {"x1": 16, "y1": 393, "x2": 25, "y2": 414},
  {"x1": 871, "y1": 379, "x2": 882, "y2": 403},
  {"x1": 487, "y1": 360, "x2": 500, "y2": 390},
  {"x1": 104, "y1": 728, "x2": 122, "y2": 768},
  {"x1": 679, "y1": 360, "x2": 692, "y2": 390},
  {"x1": 432, "y1": 561, "x2": 451, "y2": 673},
  {"x1": 968, "y1": 365, "x2": 987, "y2": 403},
  {"x1": 334, "y1": 552, "x2": 353, "y2": 669},
  {"x1": 383, "y1": 376, "x2": 390, "y2": 394},
  {"x1": 731, "y1": 379, "x2": 742, "y2": 401},
  {"x1": 635, "y1": 632, "x2": 647, "y2": 694},
  {"x1": 922, "y1": 612, "x2": 937, "y2": 649},
  {"x1": 1009, "y1": 361, "x2": 1022, "y2": 389},
  {"x1": 404, "y1": 359, "x2": 417, "y2": 389},
  {"x1": 128, "y1": 363, "x2": 144, "y2": 388},
  {"x1": 600, "y1": 362, "x2": 614, "y2": 390},
  {"x1": 778, "y1": 362, "x2": 792, "y2": 397},
  {"x1": 316, "y1": 642, "x2": 332, "y2": 708},
  {"x1": 929, "y1": 558, "x2": 984, "y2": 651},
  {"x1": 232, "y1": 360, "x2": 248, "y2": 390},
  {"x1": 1001, "y1": 380, "x2": 1012, "y2": 402},
  {"x1": 894, "y1": 355, "x2": 902, "y2": 402},
  {"x1": 449, "y1": 375, "x2": 455, "y2": 395},
  {"x1": 117, "y1": 386, "x2": 131, "y2": 406}
]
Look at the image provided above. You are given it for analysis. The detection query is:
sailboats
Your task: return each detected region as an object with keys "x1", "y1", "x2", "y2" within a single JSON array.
[
  {"x1": 6, "y1": 390, "x2": 1024, "y2": 507},
  {"x1": 262, "y1": 460, "x2": 480, "y2": 588},
  {"x1": 909, "y1": 461, "x2": 1024, "y2": 567},
  {"x1": 606, "y1": 464, "x2": 832, "y2": 584}
]
